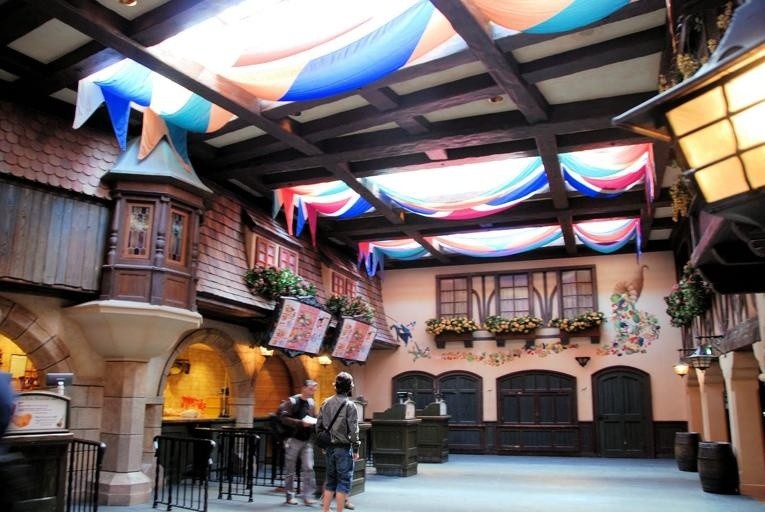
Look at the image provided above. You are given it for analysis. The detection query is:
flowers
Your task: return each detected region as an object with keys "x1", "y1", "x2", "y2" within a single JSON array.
[
  {"x1": 424, "y1": 310, "x2": 604, "y2": 336},
  {"x1": 663, "y1": 267, "x2": 715, "y2": 327},
  {"x1": 244, "y1": 265, "x2": 377, "y2": 324},
  {"x1": 656, "y1": 0, "x2": 743, "y2": 223}
]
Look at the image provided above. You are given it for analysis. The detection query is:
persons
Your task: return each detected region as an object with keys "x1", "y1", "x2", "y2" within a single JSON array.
[
  {"x1": 135, "y1": 214, "x2": 147, "y2": 249},
  {"x1": 315, "y1": 371, "x2": 360, "y2": 512},
  {"x1": 173, "y1": 215, "x2": 182, "y2": 261},
  {"x1": 0, "y1": 372, "x2": 14, "y2": 434},
  {"x1": 280, "y1": 379, "x2": 318, "y2": 505},
  {"x1": 268, "y1": 400, "x2": 288, "y2": 479}
]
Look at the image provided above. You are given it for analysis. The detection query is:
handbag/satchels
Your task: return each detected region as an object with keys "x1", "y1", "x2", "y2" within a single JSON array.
[{"x1": 314, "y1": 430, "x2": 331, "y2": 450}]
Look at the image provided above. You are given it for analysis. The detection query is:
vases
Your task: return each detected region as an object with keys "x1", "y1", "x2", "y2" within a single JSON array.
[{"x1": 435, "y1": 329, "x2": 600, "y2": 340}]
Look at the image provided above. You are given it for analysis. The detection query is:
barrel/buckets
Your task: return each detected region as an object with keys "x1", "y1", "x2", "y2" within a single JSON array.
[
  {"x1": 697, "y1": 440, "x2": 737, "y2": 494},
  {"x1": 674, "y1": 432, "x2": 700, "y2": 472}
]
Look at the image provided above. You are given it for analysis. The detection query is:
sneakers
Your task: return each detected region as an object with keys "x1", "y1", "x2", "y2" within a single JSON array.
[
  {"x1": 287, "y1": 499, "x2": 297, "y2": 505},
  {"x1": 344, "y1": 500, "x2": 354, "y2": 510},
  {"x1": 305, "y1": 499, "x2": 317, "y2": 505}
]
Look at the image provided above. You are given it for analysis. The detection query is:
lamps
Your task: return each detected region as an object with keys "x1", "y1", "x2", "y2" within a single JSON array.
[
  {"x1": 673, "y1": 334, "x2": 725, "y2": 379},
  {"x1": 613, "y1": 0, "x2": 765, "y2": 214}
]
